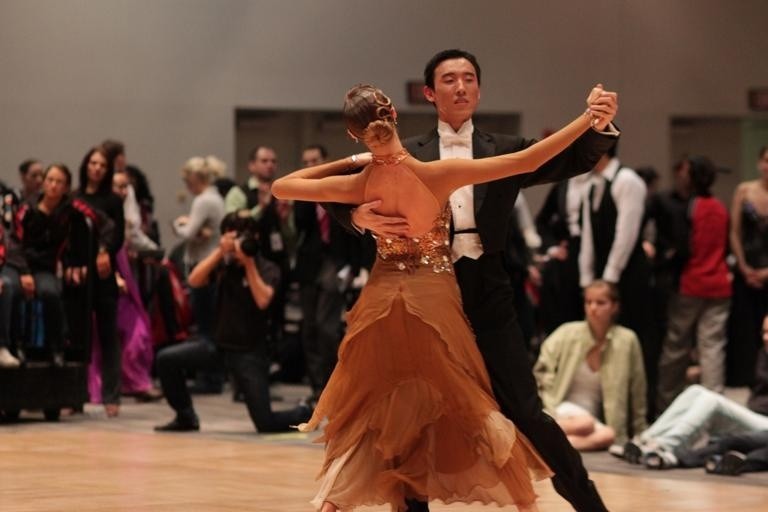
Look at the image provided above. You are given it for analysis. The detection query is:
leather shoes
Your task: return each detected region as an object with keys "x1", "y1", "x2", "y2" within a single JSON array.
[
  {"x1": 154, "y1": 414, "x2": 200, "y2": 432},
  {"x1": 136, "y1": 387, "x2": 164, "y2": 401},
  {"x1": 705, "y1": 450, "x2": 748, "y2": 473},
  {"x1": 61, "y1": 403, "x2": 119, "y2": 417}
]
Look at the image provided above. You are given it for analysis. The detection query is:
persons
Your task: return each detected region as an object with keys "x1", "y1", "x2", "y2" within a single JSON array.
[
  {"x1": 320, "y1": 50, "x2": 609, "y2": 512},
  {"x1": 499, "y1": 140, "x2": 768, "y2": 476},
  {"x1": 271, "y1": 83, "x2": 604, "y2": 512},
  {"x1": 0, "y1": 138, "x2": 377, "y2": 439}
]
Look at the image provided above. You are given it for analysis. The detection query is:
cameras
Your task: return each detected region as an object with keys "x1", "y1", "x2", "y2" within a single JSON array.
[{"x1": 230, "y1": 215, "x2": 260, "y2": 257}]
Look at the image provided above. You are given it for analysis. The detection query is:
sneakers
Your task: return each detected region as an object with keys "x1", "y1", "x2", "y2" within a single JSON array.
[{"x1": 609, "y1": 438, "x2": 680, "y2": 468}]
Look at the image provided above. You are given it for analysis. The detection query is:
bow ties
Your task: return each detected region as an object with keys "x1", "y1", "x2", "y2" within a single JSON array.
[{"x1": 441, "y1": 131, "x2": 471, "y2": 149}]
